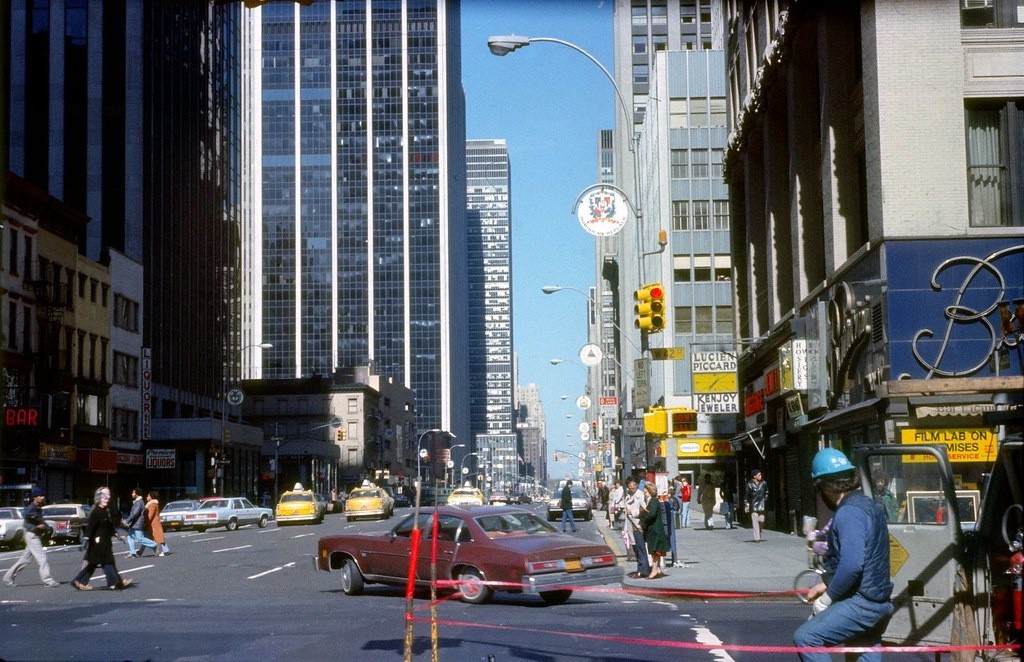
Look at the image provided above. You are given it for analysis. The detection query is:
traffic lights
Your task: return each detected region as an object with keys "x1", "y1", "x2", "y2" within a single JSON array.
[
  {"x1": 633, "y1": 285, "x2": 653, "y2": 332},
  {"x1": 592, "y1": 421, "x2": 598, "y2": 434},
  {"x1": 650, "y1": 286, "x2": 672, "y2": 332},
  {"x1": 644, "y1": 411, "x2": 668, "y2": 437},
  {"x1": 671, "y1": 410, "x2": 698, "y2": 435}
]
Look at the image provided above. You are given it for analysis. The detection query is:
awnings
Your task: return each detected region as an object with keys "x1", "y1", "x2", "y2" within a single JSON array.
[
  {"x1": 907, "y1": 394, "x2": 1022, "y2": 418},
  {"x1": 817, "y1": 397, "x2": 883, "y2": 433}
]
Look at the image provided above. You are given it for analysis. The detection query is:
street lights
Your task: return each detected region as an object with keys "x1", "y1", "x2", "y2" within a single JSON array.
[
  {"x1": 488, "y1": 35, "x2": 658, "y2": 476},
  {"x1": 417, "y1": 428, "x2": 456, "y2": 480},
  {"x1": 219, "y1": 343, "x2": 274, "y2": 495},
  {"x1": 444, "y1": 444, "x2": 465, "y2": 488},
  {"x1": 461, "y1": 452, "x2": 478, "y2": 488}
]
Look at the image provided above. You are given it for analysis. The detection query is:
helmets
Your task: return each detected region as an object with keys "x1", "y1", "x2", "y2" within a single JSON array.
[{"x1": 811, "y1": 447, "x2": 855, "y2": 478}]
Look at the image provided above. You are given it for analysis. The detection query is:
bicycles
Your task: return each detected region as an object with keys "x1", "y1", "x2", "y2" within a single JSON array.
[{"x1": 793, "y1": 535, "x2": 831, "y2": 605}]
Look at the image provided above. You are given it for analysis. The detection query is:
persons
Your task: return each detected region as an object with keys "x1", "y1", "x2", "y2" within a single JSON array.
[
  {"x1": 609, "y1": 480, "x2": 625, "y2": 530},
  {"x1": 794, "y1": 449, "x2": 893, "y2": 662},
  {"x1": 667, "y1": 475, "x2": 691, "y2": 527},
  {"x1": 122, "y1": 486, "x2": 159, "y2": 559},
  {"x1": 625, "y1": 476, "x2": 651, "y2": 578},
  {"x1": 719, "y1": 472, "x2": 737, "y2": 530},
  {"x1": 3, "y1": 488, "x2": 61, "y2": 588},
  {"x1": 72, "y1": 484, "x2": 134, "y2": 591},
  {"x1": 560, "y1": 480, "x2": 579, "y2": 532},
  {"x1": 697, "y1": 474, "x2": 717, "y2": 529},
  {"x1": 872, "y1": 468, "x2": 901, "y2": 524},
  {"x1": 639, "y1": 480, "x2": 668, "y2": 580},
  {"x1": 136, "y1": 492, "x2": 171, "y2": 556},
  {"x1": 744, "y1": 468, "x2": 769, "y2": 542}
]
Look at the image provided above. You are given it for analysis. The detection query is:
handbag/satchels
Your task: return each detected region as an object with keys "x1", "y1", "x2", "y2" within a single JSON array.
[
  {"x1": 615, "y1": 510, "x2": 625, "y2": 521},
  {"x1": 610, "y1": 507, "x2": 617, "y2": 514},
  {"x1": 719, "y1": 501, "x2": 729, "y2": 515}
]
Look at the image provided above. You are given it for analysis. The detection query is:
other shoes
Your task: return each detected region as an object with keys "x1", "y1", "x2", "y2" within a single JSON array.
[
  {"x1": 44, "y1": 580, "x2": 61, "y2": 587},
  {"x1": 154, "y1": 544, "x2": 162, "y2": 557},
  {"x1": 2, "y1": 577, "x2": 18, "y2": 588},
  {"x1": 107, "y1": 584, "x2": 122, "y2": 589},
  {"x1": 628, "y1": 571, "x2": 646, "y2": 578},
  {"x1": 119, "y1": 576, "x2": 134, "y2": 587},
  {"x1": 124, "y1": 553, "x2": 136, "y2": 558},
  {"x1": 645, "y1": 571, "x2": 664, "y2": 580},
  {"x1": 79, "y1": 583, "x2": 94, "y2": 590},
  {"x1": 71, "y1": 579, "x2": 80, "y2": 592}
]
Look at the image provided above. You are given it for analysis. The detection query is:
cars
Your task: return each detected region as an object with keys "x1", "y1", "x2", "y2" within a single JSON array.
[
  {"x1": 275, "y1": 482, "x2": 324, "y2": 526},
  {"x1": 847, "y1": 431, "x2": 1024, "y2": 662},
  {"x1": 546, "y1": 488, "x2": 593, "y2": 522},
  {"x1": 0, "y1": 506, "x2": 57, "y2": 550},
  {"x1": 311, "y1": 505, "x2": 626, "y2": 606},
  {"x1": 343, "y1": 479, "x2": 546, "y2": 522},
  {"x1": 41, "y1": 503, "x2": 91, "y2": 544},
  {"x1": 183, "y1": 497, "x2": 274, "y2": 534},
  {"x1": 159, "y1": 497, "x2": 226, "y2": 532}
]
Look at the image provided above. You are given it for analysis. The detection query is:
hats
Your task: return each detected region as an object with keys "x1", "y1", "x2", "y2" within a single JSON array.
[{"x1": 30, "y1": 487, "x2": 44, "y2": 499}]
